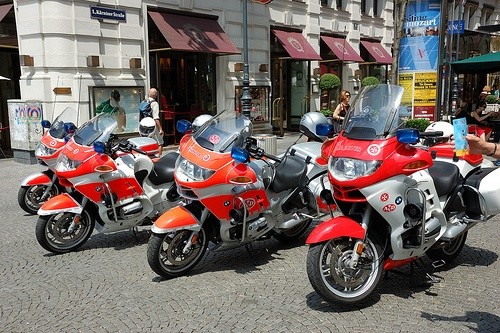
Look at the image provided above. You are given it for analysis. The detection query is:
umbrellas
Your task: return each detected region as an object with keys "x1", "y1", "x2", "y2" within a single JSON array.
[{"x1": 451, "y1": 50, "x2": 500, "y2": 75}]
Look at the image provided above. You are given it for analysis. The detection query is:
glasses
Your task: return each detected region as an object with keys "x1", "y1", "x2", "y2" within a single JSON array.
[{"x1": 346, "y1": 95, "x2": 350, "y2": 98}]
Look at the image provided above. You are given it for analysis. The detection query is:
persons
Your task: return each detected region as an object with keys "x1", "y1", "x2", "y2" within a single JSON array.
[
  {"x1": 140, "y1": 88, "x2": 164, "y2": 158},
  {"x1": 96, "y1": 89, "x2": 126, "y2": 132},
  {"x1": 333, "y1": 90, "x2": 353, "y2": 133},
  {"x1": 447, "y1": 134, "x2": 500, "y2": 160},
  {"x1": 406, "y1": 28, "x2": 413, "y2": 37},
  {"x1": 425, "y1": 28, "x2": 439, "y2": 36},
  {"x1": 466, "y1": 102, "x2": 494, "y2": 142}
]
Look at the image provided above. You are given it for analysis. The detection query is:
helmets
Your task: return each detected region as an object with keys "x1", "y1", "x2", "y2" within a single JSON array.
[
  {"x1": 300, "y1": 112, "x2": 329, "y2": 143},
  {"x1": 424, "y1": 122, "x2": 454, "y2": 142},
  {"x1": 192, "y1": 114, "x2": 218, "y2": 132},
  {"x1": 139, "y1": 117, "x2": 156, "y2": 137}
]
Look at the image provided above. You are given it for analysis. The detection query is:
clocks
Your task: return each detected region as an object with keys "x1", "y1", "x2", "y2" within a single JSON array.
[{"x1": 296, "y1": 72, "x2": 303, "y2": 80}]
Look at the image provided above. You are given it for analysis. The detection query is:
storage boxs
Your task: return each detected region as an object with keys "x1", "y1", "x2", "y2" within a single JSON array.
[
  {"x1": 308, "y1": 174, "x2": 336, "y2": 212},
  {"x1": 464, "y1": 167, "x2": 500, "y2": 218}
]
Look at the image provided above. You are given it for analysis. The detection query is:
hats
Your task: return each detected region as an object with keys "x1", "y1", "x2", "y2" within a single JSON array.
[{"x1": 111, "y1": 90, "x2": 120, "y2": 101}]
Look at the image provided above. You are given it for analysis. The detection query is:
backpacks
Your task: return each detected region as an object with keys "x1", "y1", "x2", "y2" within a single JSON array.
[{"x1": 139, "y1": 98, "x2": 157, "y2": 118}]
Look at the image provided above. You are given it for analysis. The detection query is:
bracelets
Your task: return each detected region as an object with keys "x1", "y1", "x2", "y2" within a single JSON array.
[{"x1": 487, "y1": 143, "x2": 497, "y2": 156}]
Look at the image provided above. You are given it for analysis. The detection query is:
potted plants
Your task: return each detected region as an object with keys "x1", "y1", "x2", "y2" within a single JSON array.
[
  {"x1": 484, "y1": 95, "x2": 500, "y2": 112},
  {"x1": 317, "y1": 73, "x2": 341, "y2": 125}
]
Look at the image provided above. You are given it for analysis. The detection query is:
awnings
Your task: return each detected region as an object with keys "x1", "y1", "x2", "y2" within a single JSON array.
[
  {"x1": 0, "y1": 4, "x2": 14, "y2": 22},
  {"x1": 271, "y1": 28, "x2": 322, "y2": 60},
  {"x1": 149, "y1": 11, "x2": 242, "y2": 56},
  {"x1": 359, "y1": 40, "x2": 393, "y2": 66},
  {"x1": 319, "y1": 35, "x2": 364, "y2": 69}
]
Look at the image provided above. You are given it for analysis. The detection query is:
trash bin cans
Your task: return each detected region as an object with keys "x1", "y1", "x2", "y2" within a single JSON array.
[{"x1": 250, "y1": 134, "x2": 277, "y2": 156}]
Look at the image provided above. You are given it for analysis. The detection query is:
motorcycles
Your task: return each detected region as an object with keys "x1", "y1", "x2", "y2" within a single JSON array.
[
  {"x1": 16, "y1": 105, "x2": 184, "y2": 254},
  {"x1": 301, "y1": 82, "x2": 500, "y2": 307},
  {"x1": 145, "y1": 109, "x2": 339, "y2": 279}
]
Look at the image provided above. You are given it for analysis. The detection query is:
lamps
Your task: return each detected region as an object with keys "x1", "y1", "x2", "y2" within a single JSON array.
[
  {"x1": 87, "y1": 56, "x2": 99, "y2": 66},
  {"x1": 130, "y1": 58, "x2": 142, "y2": 68},
  {"x1": 234, "y1": 63, "x2": 243, "y2": 71},
  {"x1": 19, "y1": 55, "x2": 33, "y2": 66},
  {"x1": 355, "y1": 70, "x2": 361, "y2": 76},
  {"x1": 259, "y1": 64, "x2": 268, "y2": 71},
  {"x1": 313, "y1": 68, "x2": 320, "y2": 75}
]
